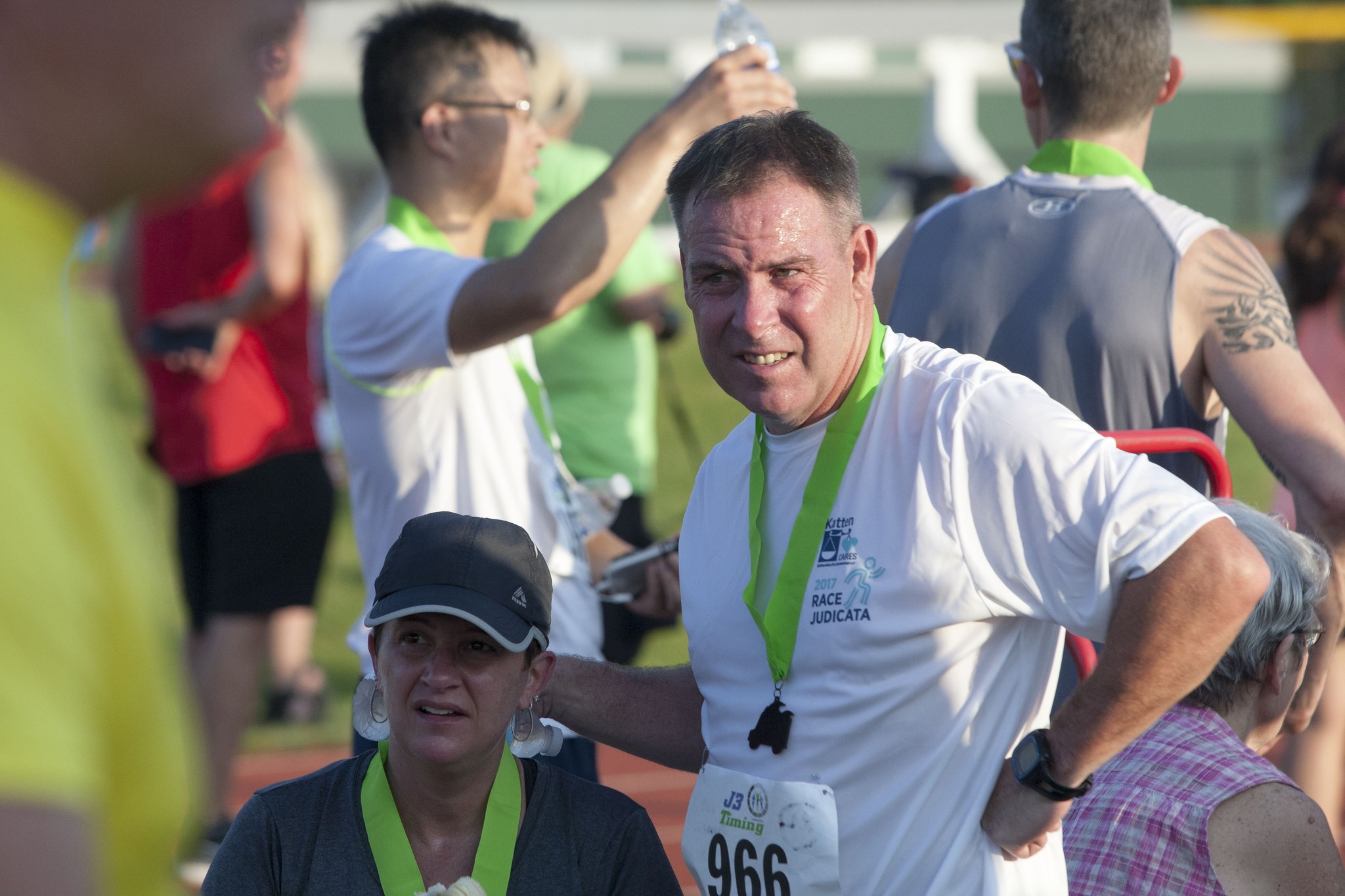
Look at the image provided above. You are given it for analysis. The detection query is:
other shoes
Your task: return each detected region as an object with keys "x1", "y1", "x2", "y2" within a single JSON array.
[
  {"x1": 265, "y1": 686, "x2": 325, "y2": 726},
  {"x1": 179, "y1": 822, "x2": 231, "y2": 887}
]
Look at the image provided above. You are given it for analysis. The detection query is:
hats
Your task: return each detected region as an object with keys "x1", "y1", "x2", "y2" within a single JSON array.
[{"x1": 363, "y1": 510, "x2": 551, "y2": 653}]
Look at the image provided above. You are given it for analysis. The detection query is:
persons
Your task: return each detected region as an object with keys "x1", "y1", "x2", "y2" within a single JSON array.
[
  {"x1": 200, "y1": 511, "x2": 686, "y2": 895},
  {"x1": 108, "y1": 33, "x2": 345, "y2": 888},
  {"x1": 1274, "y1": 127, "x2": 1345, "y2": 531},
  {"x1": 0, "y1": 0, "x2": 306, "y2": 896},
  {"x1": 324, "y1": 3, "x2": 799, "y2": 783},
  {"x1": 536, "y1": 110, "x2": 1272, "y2": 895},
  {"x1": 871, "y1": 3, "x2": 1345, "y2": 715},
  {"x1": 1062, "y1": 499, "x2": 1344, "y2": 894}
]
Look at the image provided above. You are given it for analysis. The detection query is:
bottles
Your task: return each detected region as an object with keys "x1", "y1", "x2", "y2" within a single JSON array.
[
  {"x1": 351, "y1": 671, "x2": 564, "y2": 760},
  {"x1": 576, "y1": 472, "x2": 634, "y2": 530},
  {"x1": 711, "y1": 0, "x2": 781, "y2": 75}
]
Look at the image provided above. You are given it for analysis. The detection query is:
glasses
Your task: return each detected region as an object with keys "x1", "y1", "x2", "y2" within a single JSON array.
[
  {"x1": 443, "y1": 94, "x2": 531, "y2": 124},
  {"x1": 1004, "y1": 40, "x2": 1044, "y2": 95},
  {"x1": 1276, "y1": 612, "x2": 1323, "y2": 648}
]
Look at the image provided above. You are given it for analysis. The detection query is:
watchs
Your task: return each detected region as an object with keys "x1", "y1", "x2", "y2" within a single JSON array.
[{"x1": 1012, "y1": 729, "x2": 1093, "y2": 801}]
